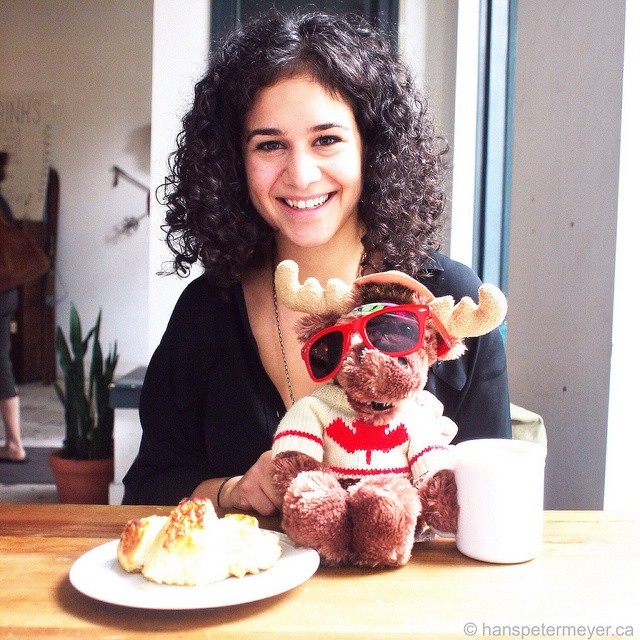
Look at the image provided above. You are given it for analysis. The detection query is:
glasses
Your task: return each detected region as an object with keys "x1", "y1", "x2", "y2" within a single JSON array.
[{"x1": 301, "y1": 303, "x2": 451, "y2": 383}]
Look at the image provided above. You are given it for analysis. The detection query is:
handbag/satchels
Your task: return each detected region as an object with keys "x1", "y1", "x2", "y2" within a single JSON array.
[{"x1": 2, "y1": 221, "x2": 49, "y2": 291}]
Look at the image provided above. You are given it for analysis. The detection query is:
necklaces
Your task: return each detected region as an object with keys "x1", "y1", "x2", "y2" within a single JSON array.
[{"x1": 267, "y1": 249, "x2": 299, "y2": 406}]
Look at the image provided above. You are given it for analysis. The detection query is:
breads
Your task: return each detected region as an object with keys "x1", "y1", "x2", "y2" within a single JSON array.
[
  {"x1": 150, "y1": 498, "x2": 221, "y2": 586},
  {"x1": 221, "y1": 515, "x2": 284, "y2": 577},
  {"x1": 116, "y1": 515, "x2": 165, "y2": 573}
]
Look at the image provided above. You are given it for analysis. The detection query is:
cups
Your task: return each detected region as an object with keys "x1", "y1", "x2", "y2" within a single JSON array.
[{"x1": 452, "y1": 436, "x2": 547, "y2": 565}]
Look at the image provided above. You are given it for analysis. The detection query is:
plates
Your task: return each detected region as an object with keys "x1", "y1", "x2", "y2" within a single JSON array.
[{"x1": 68, "y1": 526, "x2": 321, "y2": 611}]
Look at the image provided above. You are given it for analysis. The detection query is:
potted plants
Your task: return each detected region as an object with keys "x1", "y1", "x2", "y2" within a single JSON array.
[{"x1": 48, "y1": 302, "x2": 121, "y2": 503}]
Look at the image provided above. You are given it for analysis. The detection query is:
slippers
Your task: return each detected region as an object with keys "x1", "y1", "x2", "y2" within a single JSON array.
[{"x1": 3, "y1": 457, "x2": 30, "y2": 463}]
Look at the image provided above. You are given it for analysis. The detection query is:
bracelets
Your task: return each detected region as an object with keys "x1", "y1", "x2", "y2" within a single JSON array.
[{"x1": 216, "y1": 473, "x2": 242, "y2": 508}]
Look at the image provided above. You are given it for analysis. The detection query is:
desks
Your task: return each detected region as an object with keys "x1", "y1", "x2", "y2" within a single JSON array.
[{"x1": 0, "y1": 503, "x2": 609, "y2": 640}]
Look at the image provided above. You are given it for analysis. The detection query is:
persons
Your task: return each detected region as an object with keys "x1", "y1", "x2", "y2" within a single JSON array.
[
  {"x1": 1, "y1": 151, "x2": 33, "y2": 462},
  {"x1": 121, "y1": 12, "x2": 516, "y2": 517}
]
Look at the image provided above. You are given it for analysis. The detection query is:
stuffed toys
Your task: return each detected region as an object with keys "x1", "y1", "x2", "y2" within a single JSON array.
[{"x1": 270, "y1": 258, "x2": 507, "y2": 566}]
